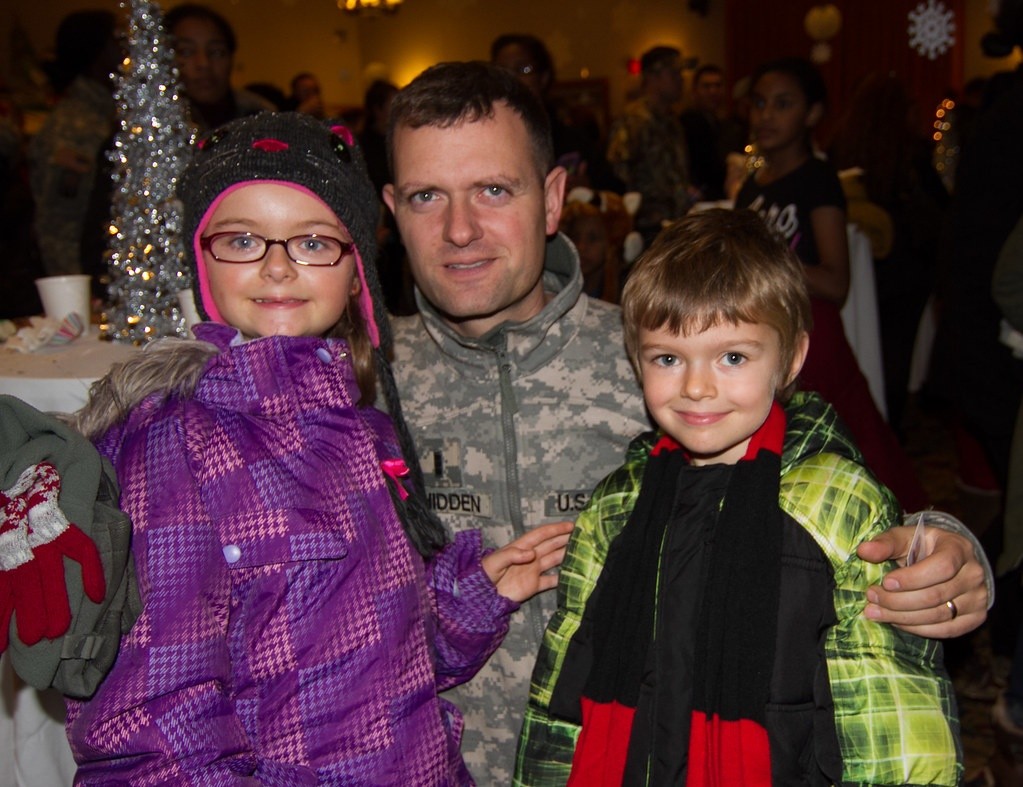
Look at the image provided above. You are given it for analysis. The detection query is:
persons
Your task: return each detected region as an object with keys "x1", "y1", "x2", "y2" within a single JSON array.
[
  {"x1": 0, "y1": 0, "x2": 1023, "y2": 516},
  {"x1": 66, "y1": 113, "x2": 576, "y2": 787},
  {"x1": 510, "y1": 206, "x2": 965, "y2": 787},
  {"x1": 370, "y1": 61, "x2": 994, "y2": 787}
]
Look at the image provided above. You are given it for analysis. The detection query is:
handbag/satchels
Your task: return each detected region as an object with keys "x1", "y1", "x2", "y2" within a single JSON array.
[{"x1": 0, "y1": 392, "x2": 145, "y2": 696}]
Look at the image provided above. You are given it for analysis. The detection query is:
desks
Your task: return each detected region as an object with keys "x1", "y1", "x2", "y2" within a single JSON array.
[{"x1": 0, "y1": 311, "x2": 145, "y2": 413}]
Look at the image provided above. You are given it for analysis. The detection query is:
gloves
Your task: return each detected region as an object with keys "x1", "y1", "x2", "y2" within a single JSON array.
[{"x1": 0, "y1": 461, "x2": 106, "y2": 655}]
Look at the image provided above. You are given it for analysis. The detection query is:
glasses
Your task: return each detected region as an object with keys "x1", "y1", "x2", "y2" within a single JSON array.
[{"x1": 201, "y1": 231, "x2": 355, "y2": 268}]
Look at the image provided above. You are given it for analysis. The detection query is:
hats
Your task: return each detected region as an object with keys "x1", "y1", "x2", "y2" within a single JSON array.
[{"x1": 174, "y1": 110, "x2": 427, "y2": 504}]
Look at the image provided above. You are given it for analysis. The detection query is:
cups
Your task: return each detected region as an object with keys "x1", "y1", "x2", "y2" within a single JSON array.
[
  {"x1": 36, "y1": 275, "x2": 92, "y2": 336},
  {"x1": 177, "y1": 289, "x2": 202, "y2": 339}
]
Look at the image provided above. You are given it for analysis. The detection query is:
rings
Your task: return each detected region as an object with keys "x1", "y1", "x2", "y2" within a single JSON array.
[{"x1": 946, "y1": 600, "x2": 958, "y2": 620}]
